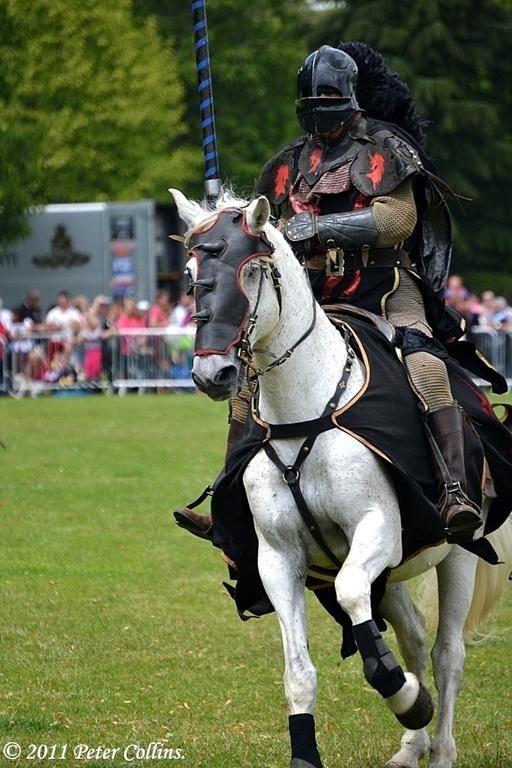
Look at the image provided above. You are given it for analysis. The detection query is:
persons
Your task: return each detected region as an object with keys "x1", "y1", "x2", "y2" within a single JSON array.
[
  {"x1": 175, "y1": 43, "x2": 483, "y2": 544},
  {"x1": 444, "y1": 274, "x2": 512, "y2": 380},
  {"x1": 0, "y1": 286, "x2": 197, "y2": 399}
]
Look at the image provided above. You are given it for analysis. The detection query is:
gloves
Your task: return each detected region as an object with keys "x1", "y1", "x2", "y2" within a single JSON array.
[{"x1": 277, "y1": 213, "x2": 319, "y2": 261}]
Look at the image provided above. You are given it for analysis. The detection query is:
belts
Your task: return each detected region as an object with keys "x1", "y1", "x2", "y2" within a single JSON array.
[{"x1": 299, "y1": 249, "x2": 415, "y2": 276}]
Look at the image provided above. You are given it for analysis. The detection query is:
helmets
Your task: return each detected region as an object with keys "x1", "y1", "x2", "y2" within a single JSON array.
[{"x1": 293, "y1": 44, "x2": 363, "y2": 135}]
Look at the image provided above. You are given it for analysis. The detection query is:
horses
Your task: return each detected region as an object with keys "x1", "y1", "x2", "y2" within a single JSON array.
[{"x1": 167, "y1": 187, "x2": 512, "y2": 768}]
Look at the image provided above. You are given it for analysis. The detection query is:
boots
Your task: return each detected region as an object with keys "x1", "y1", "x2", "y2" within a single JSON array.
[
  {"x1": 171, "y1": 412, "x2": 246, "y2": 569},
  {"x1": 426, "y1": 403, "x2": 479, "y2": 545}
]
